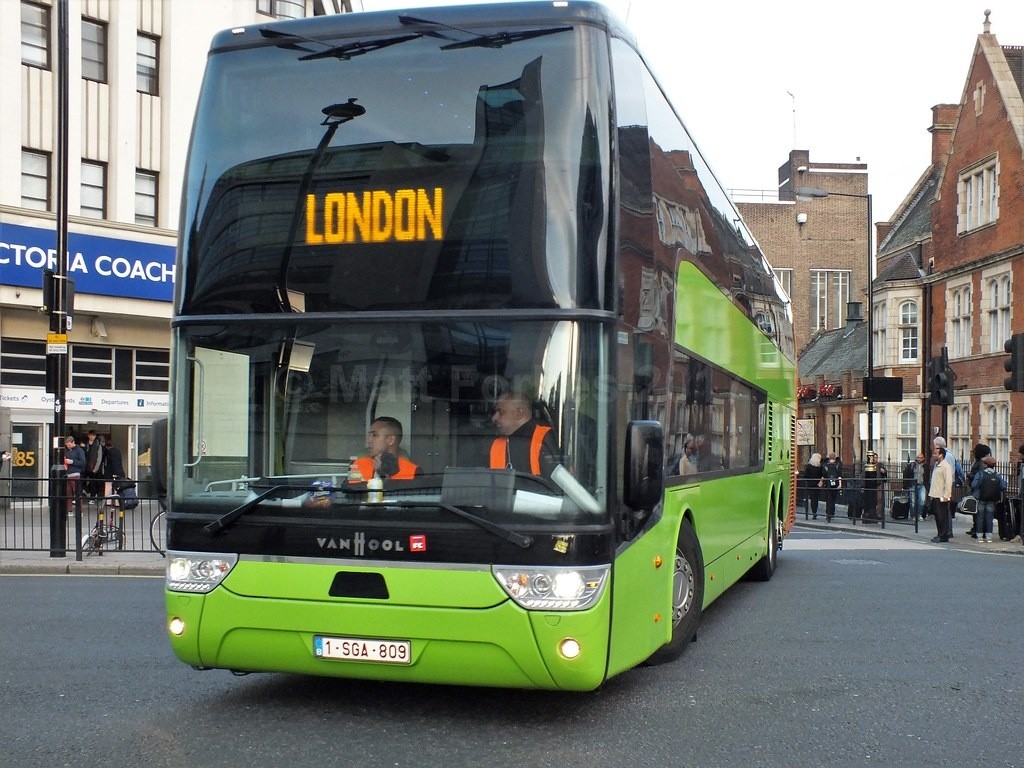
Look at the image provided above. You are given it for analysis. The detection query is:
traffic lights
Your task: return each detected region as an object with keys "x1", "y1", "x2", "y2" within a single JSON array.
[
  {"x1": 927, "y1": 355, "x2": 941, "y2": 404},
  {"x1": 933, "y1": 371, "x2": 954, "y2": 405},
  {"x1": 1003, "y1": 333, "x2": 1024, "y2": 393}
]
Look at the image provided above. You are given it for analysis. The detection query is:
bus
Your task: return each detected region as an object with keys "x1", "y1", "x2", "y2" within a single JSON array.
[{"x1": 162, "y1": 0, "x2": 799, "y2": 690}]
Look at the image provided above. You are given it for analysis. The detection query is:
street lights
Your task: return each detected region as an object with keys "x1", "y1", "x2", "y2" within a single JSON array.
[{"x1": 795, "y1": 185, "x2": 885, "y2": 525}]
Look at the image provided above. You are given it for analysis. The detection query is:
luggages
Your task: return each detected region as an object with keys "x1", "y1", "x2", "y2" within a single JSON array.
[
  {"x1": 996, "y1": 488, "x2": 1012, "y2": 541},
  {"x1": 889, "y1": 490, "x2": 910, "y2": 520},
  {"x1": 1005, "y1": 496, "x2": 1022, "y2": 535},
  {"x1": 112, "y1": 474, "x2": 140, "y2": 510},
  {"x1": 848, "y1": 491, "x2": 863, "y2": 520}
]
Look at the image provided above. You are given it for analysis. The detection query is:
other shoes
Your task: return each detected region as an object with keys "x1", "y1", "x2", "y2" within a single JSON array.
[
  {"x1": 986, "y1": 537, "x2": 992, "y2": 543},
  {"x1": 89, "y1": 500, "x2": 95, "y2": 504},
  {"x1": 967, "y1": 528, "x2": 977, "y2": 538},
  {"x1": 946, "y1": 534, "x2": 953, "y2": 538},
  {"x1": 978, "y1": 537, "x2": 984, "y2": 542},
  {"x1": 72, "y1": 499, "x2": 76, "y2": 505},
  {"x1": 931, "y1": 536, "x2": 949, "y2": 543},
  {"x1": 913, "y1": 515, "x2": 924, "y2": 522}
]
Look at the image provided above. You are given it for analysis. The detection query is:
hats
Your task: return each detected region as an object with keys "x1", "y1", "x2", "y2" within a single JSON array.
[{"x1": 981, "y1": 455, "x2": 996, "y2": 467}]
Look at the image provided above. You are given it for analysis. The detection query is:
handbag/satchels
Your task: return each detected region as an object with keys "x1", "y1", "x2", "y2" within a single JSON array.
[
  {"x1": 956, "y1": 494, "x2": 978, "y2": 515},
  {"x1": 952, "y1": 483, "x2": 972, "y2": 504},
  {"x1": 113, "y1": 477, "x2": 136, "y2": 491},
  {"x1": 879, "y1": 463, "x2": 887, "y2": 483}
]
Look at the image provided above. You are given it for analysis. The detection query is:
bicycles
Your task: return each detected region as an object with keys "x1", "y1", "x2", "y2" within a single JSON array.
[
  {"x1": 82, "y1": 491, "x2": 125, "y2": 558},
  {"x1": 148, "y1": 510, "x2": 168, "y2": 559}
]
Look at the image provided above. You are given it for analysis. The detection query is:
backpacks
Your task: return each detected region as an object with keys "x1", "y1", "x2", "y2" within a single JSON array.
[
  {"x1": 979, "y1": 470, "x2": 1001, "y2": 501},
  {"x1": 824, "y1": 462, "x2": 839, "y2": 488},
  {"x1": 903, "y1": 462, "x2": 915, "y2": 490},
  {"x1": 955, "y1": 460, "x2": 965, "y2": 483}
]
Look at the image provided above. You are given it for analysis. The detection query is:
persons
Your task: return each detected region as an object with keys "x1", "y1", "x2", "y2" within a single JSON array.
[
  {"x1": 672, "y1": 434, "x2": 709, "y2": 475},
  {"x1": 805, "y1": 436, "x2": 1024, "y2": 544},
  {"x1": 339, "y1": 416, "x2": 426, "y2": 485},
  {"x1": 476, "y1": 390, "x2": 569, "y2": 494},
  {"x1": 64, "y1": 430, "x2": 126, "y2": 516}
]
